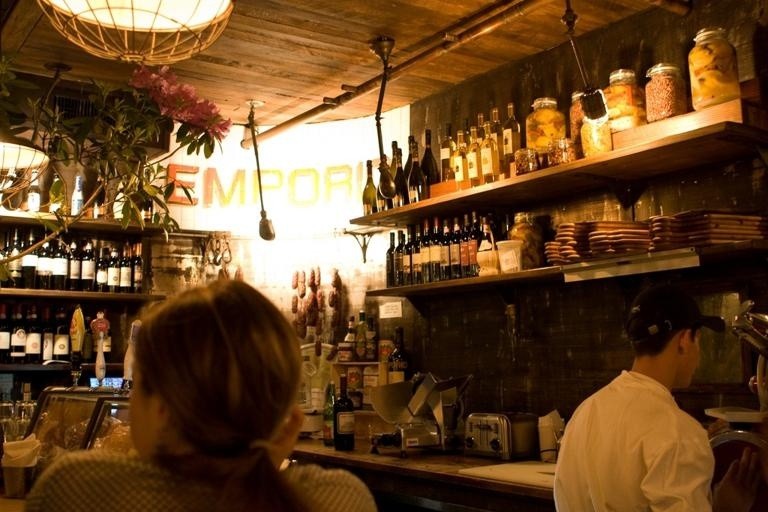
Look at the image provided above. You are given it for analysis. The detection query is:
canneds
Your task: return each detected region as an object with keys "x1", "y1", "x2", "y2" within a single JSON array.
[{"x1": 526, "y1": 27, "x2": 741, "y2": 156}]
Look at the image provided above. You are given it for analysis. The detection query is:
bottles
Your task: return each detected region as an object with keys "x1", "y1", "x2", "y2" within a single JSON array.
[
  {"x1": 512, "y1": 147, "x2": 541, "y2": 177},
  {"x1": 14, "y1": 383, "x2": 38, "y2": 440},
  {"x1": 362, "y1": 103, "x2": 520, "y2": 215},
  {"x1": 323, "y1": 382, "x2": 336, "y2": 446},
  {"x1": 334, "y1": 374, "x2": 356, "y2": 450},
  {"x1": 0, "y1": 301, "x2": 112, "y2": 363},
  {"x1": 568, "y1": 89, "x2": 588, "y2": 143},
  {"x1": 336, "y1": 310, "x2": 378, "y2": 363},
  {"x1": 642, "y1": 62, "x2": 688, "y2": 124},
  {"x1": 544, "y1": 136, "x2": 577, "y2": 168},
  {"x1": 0, "y1": 227, "x2": 147, "y2": 293},
  {"x1": 384, "y1": 209, "x2": 512, "y2": 288},
  {"x1": 386, "y1": 325, "x2": 413, "y2": 381},
  {"x1": 1, "y1": 167, "x2": 155, "y2": 226}
]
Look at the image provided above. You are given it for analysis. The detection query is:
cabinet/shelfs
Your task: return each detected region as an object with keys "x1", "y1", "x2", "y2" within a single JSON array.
[
  {"x1": 0, "y1": 210, "x2": 166, "y2": 370},
  {"x1": 350, "y1": 98, "x2": 768, "y2": 296}
]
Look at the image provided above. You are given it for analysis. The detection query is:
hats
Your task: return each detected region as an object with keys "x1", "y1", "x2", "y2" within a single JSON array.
[{"x1": 626, "y1": 285, "x2": 724, "y2": 344}]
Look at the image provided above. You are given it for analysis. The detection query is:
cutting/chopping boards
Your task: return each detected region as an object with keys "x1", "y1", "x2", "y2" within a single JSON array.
[{"x1": 543, "y1": 206, "x2": 767, "y2": 265}]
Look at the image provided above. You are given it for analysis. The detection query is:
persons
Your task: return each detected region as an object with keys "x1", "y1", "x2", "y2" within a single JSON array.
[
  {"x1": 22, "y1": 281, "x2": 378, "y2": 512},
  {"x1": 553, "y1": 285, "x2": 761, "y2": 512}
]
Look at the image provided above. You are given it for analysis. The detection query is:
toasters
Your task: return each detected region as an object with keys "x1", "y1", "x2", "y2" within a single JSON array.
[{"x1": 460, "y1": 409, "x2": 539, "y2": 462}]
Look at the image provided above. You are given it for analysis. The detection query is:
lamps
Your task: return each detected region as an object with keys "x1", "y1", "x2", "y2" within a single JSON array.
[
  {"x1": 248, "y1": 101, "x2": 275, "y2": 241},
  {"x1": 38, "y1": 0, "x2": 232, "y2": 66},
  {"x1": 561, "y1": 0, "x2": 609, "y2": 124},
  {"x1": 369, "y1": 38, "x2": 396, "y2": 199}
]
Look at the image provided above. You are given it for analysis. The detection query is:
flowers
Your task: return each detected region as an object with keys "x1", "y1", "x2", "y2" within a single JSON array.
[{"x1": 0, "y1": 56, "x2": 233, "y2": 282}]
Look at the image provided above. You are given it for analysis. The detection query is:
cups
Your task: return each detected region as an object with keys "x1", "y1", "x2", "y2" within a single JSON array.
[
  {"x1": 494, "y1": 239, "x2": 523, "y2": 272},
  {"x1": 0, "y1": 401, "x2": 18, "y2": 444}
]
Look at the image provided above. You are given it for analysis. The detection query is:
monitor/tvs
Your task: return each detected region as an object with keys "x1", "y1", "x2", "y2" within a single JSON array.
[
  {"x1": 87, "y1": 374, "x2": 124, "y2": 416},
  {"x1": 12, "y1": 364, "x2": 72, "y2": 399}
]
